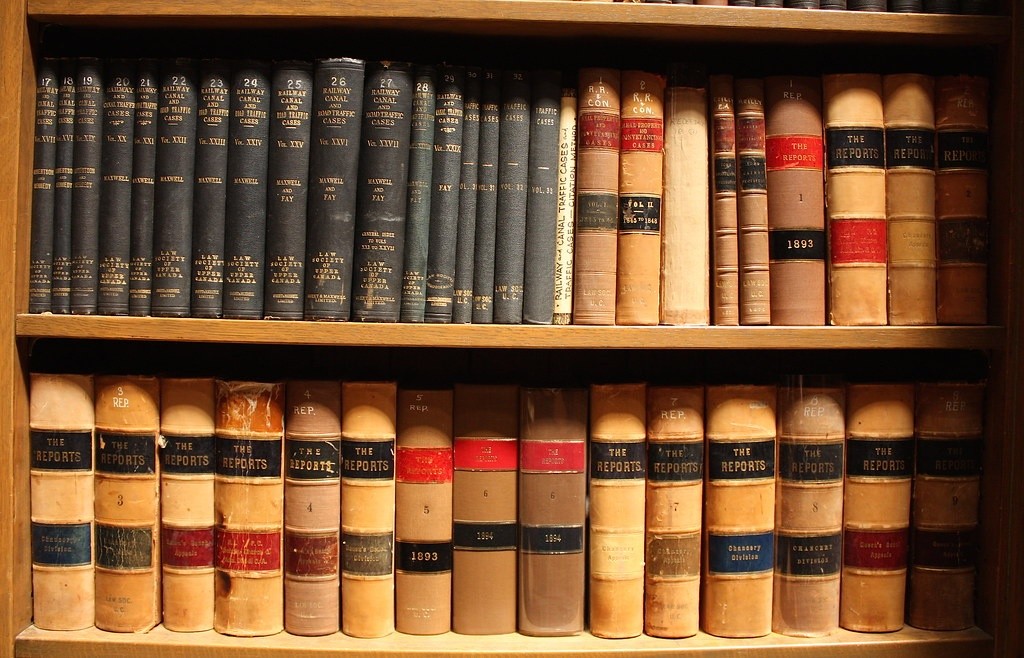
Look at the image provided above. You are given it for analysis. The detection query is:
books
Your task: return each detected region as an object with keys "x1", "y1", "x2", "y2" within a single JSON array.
[
  {"x1": 31, "y1": 48, "x2": 997, "y2": 329},
  {"x1": 610, "y1": 0, "x2": 1011, "y2": 15},
  {"x1": 28, "y1": 369, "x2": 981, "y2": 631}
]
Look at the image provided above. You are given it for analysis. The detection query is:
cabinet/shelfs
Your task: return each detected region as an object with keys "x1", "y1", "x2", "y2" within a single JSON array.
[{"x1": 0, "y1": 0, "x2": 1024, "y2": 658}]
